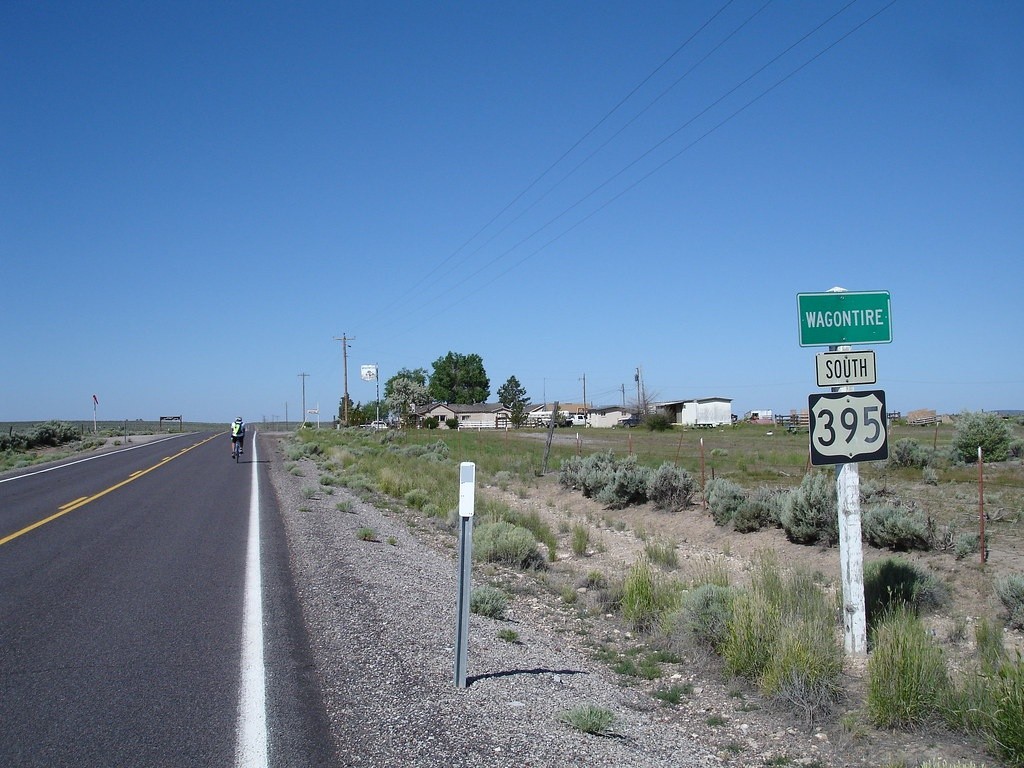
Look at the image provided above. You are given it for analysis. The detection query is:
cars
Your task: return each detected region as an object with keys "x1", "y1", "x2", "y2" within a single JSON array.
[{"x1": 618, "y1": 414, "x2": 645, "y2": 429}]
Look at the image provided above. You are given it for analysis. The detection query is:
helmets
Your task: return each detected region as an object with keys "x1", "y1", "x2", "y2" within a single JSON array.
[{"x1": 236, "y1": 417, "x2": 242, "y2": 421}]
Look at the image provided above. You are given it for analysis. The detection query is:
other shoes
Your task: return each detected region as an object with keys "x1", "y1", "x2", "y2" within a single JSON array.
[
  {"x1": 239, "y1": 448, "x2": 243, "y2": 453},
  {"x1": 231, "y1": 452, "x2": 235, "y2": 459}
]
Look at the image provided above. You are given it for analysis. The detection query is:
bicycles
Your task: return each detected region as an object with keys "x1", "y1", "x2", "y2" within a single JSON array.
[{"x1": 231, "y1": 428, "x2": 244, "y2": 463}]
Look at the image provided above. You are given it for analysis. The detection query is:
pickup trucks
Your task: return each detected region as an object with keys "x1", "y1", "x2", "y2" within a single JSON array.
[{"x1": 568, "y1": 415, "x2": 589, "y2": 428}]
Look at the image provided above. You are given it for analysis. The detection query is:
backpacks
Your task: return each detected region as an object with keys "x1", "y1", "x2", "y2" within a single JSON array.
[{"x1": 234, "y1": 422, "x2": 244, "y2": 434}]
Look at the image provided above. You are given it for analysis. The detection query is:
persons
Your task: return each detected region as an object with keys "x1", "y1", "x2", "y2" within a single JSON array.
[{"x1": 230, "y1": 417, "x2": 245, "y2": 459}]
